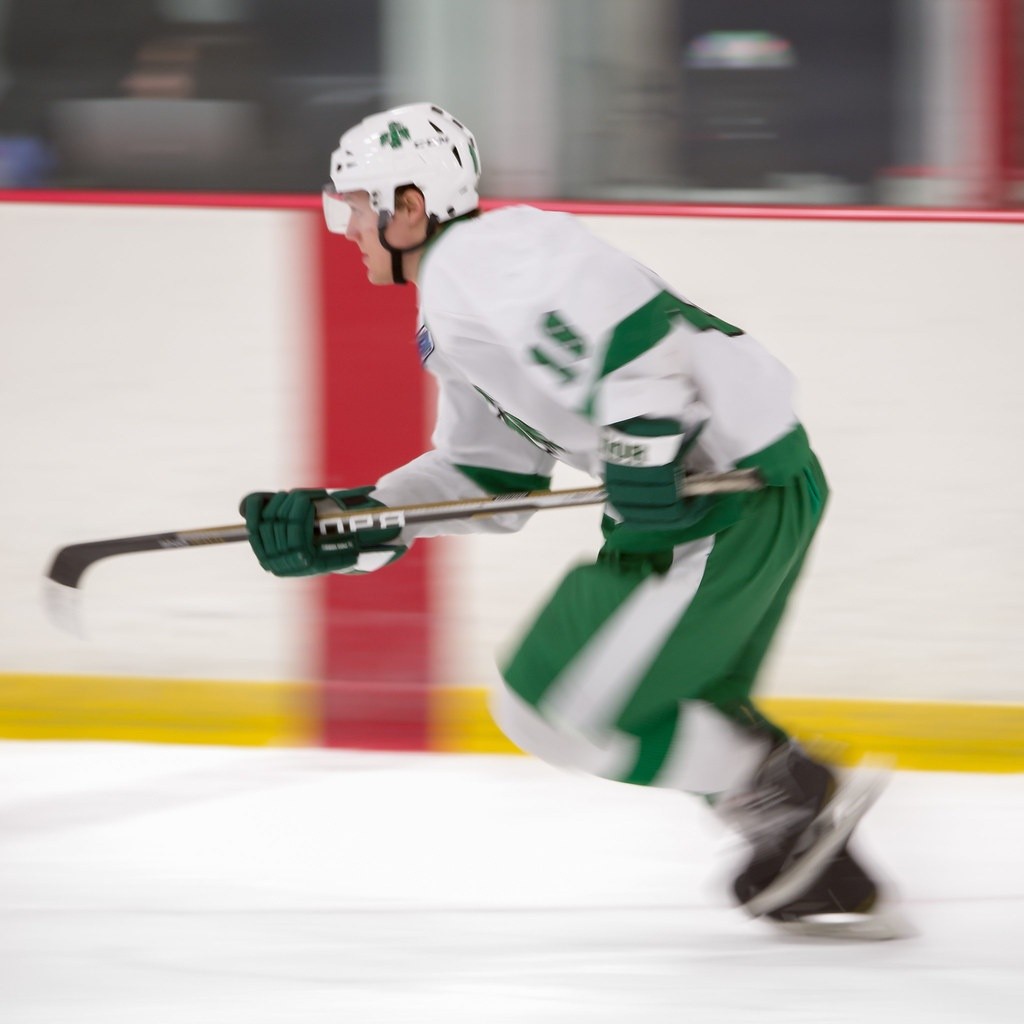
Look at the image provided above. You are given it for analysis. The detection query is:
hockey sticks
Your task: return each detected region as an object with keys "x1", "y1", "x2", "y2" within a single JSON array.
[{"x1": 42, "y1": 466, "x2": 764, "y2": 634}]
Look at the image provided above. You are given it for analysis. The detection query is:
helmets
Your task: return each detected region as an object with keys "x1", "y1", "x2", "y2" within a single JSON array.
[{"x1": 329, "y1": 101, "x2": 482, "y2": 227}]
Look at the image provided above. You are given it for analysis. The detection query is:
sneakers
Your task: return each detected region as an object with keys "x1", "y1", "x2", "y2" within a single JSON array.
[{"x1": 712, "y1": 739, "x2": 887, "y2": 924}]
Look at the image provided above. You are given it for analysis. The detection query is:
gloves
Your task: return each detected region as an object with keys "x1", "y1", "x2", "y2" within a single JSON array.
[
  {"x1": 239, "y1": 483, "x2": 409, "y2": 578},
  {"x1": 596, "y1": 413, "x2": 711, "y2": 574}
]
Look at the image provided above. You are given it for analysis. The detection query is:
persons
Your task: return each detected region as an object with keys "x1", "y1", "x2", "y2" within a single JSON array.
[{"x1": 237, "y1": 98, "x2": 881, "y2": 920}]
